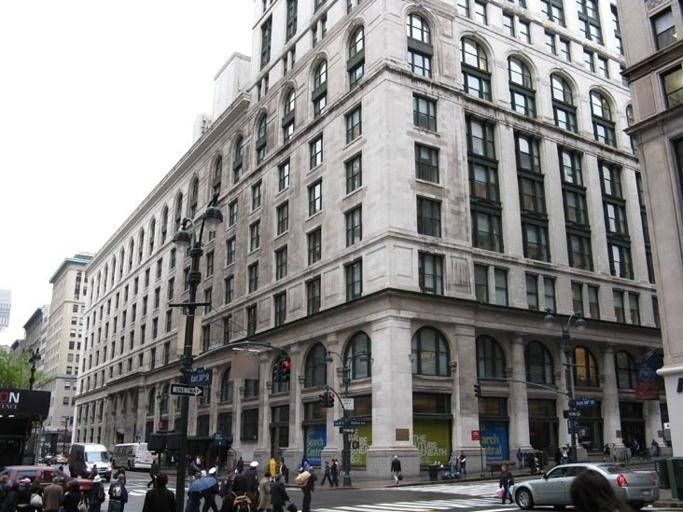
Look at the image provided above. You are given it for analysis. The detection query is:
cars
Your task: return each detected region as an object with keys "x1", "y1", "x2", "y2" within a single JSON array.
[{"x1": 511, "y1": 462, "x2": 660, "y2": 509}]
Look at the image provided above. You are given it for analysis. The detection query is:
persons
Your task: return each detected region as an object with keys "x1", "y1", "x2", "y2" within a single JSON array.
[
  {"x1": 0, "y1": 451, "x2": 340, "y2": 512},
  {"x1": 448, "y1": 438, "x2": 659, "y2": 511},
  {"x1": 390, "y1": 455, "x2": 401, "y2": 484}
]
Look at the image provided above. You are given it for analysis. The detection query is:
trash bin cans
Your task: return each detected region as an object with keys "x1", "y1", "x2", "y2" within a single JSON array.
[
  {"x1": 429, "y1": 464, "x2": 437, "y2": 481},
  {"x1": 654, "y1": 458, "x2": 670, "y2": 489}
]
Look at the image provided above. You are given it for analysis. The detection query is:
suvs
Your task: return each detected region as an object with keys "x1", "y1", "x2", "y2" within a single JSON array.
[{"x1": 0, "y1": 466, "x2": 105, "y2": 511}]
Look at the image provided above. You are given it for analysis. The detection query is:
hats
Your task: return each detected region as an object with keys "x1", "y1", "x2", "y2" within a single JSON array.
[
  {"x1": 209, "y1": 467, "x2": 216, "y2": 474},
  {"x1": 250, "y1": 461, "x2": 258, "y2": 467},
  {"x1": 93, "y1": 475, "x2": 102, "y2": 483}
]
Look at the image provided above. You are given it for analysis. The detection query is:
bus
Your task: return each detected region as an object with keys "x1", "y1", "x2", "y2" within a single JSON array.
[{"x1": 113, "y1": 442, "x2": 153, "y2": 470}]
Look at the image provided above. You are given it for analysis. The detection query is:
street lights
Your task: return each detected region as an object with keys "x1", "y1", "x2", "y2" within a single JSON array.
[
  {"x1": 539, "y1": 304, "x2": 587, "y2": 461},
  {"x1": 170, "y1": 205, "x2": 223, "y2": 510},
  {"x1": 8, "y1": 339, "x2": 42, "y2": 435}
]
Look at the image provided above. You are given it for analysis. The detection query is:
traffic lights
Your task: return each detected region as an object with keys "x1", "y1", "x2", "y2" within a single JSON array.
[
  {"x1": 473, "y1": 383, "x2": 481, "y2": 397},
  {"x1": 274, "y1": 352, "x2": 290, "y2": 383},
  {"x1": 317, "y1": 390, "x2": 334, "y2": 408}
]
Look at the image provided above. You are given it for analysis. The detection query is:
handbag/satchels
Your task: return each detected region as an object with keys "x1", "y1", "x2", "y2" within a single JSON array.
[
  {"x1": 77, "y1": 497, "x2": 90, "y2": 511},
  {"x1": 30, "y1": 493, "x2": 43, "y2": 507},
  {"x1": 399, "y1": 475, "x2": 403, "y2": 480},
  {"x1": 294, "y1": 471, "x2": 311, "y2": 487},
  {"x1": 231, "y1": 491, "x2": 252, "y2": 512}
]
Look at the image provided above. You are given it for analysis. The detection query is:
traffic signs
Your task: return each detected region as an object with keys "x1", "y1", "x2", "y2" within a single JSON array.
[
  {"x1": 573, "y1": 398, "x2": 595, "y2": 409},
  {"x1": 170, "y1": 383, "x2": 203, "y2": 397},
  {"x1": 350, "y1": 419, "x2": 367, "y2": 425},
  {"x1": 333, "y1": 420, "x2": 346, "y2": 426},
  {"x1": 187, "y1": 367, "x2": 212, "y2": 386}
]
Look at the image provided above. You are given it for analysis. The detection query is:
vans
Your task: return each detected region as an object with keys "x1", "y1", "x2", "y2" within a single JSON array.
[{"x1": 69, "y1": 443, "x2": 111, "y2": 482}]
